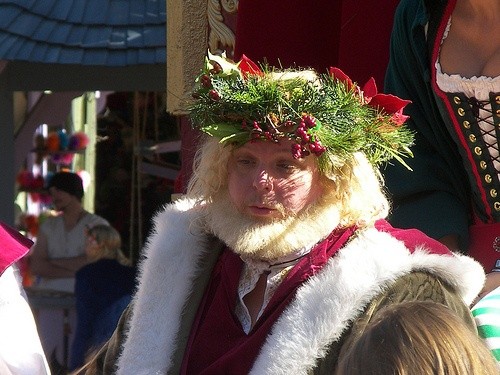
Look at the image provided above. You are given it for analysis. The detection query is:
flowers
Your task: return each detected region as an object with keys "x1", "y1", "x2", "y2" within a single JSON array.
[{"x1": 170, "y1": 48, "x2": 417, "y2": 171}]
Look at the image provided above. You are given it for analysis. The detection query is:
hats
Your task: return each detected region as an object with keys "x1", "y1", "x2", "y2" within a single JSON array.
[{"x1": 48, "y1": 172, "x2": 83, "y2": 196}]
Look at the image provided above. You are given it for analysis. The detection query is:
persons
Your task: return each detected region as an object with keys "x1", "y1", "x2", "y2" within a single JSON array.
[
  {"x1": 377, "y1": 0, "x2": 500, "y2": 309},
  {"x1": 0, "y1": 48, "x2": 500, "y2": 375}
]
